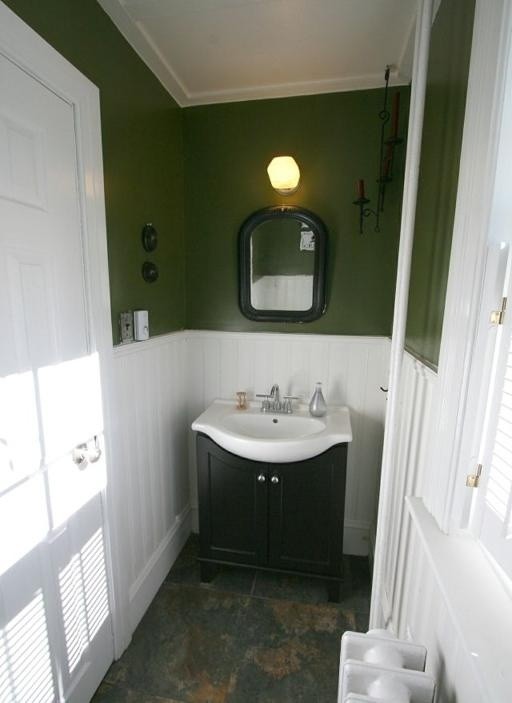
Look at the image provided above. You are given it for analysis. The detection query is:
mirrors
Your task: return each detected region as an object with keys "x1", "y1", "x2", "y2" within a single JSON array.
[{"x1": 236, "y1": 204, "x2": 328, "y2": 327}]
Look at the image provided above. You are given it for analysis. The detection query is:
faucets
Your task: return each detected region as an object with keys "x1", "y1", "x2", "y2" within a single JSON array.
[{"x1": 270, "y1": 384, "x2": 282, "y2": 412}]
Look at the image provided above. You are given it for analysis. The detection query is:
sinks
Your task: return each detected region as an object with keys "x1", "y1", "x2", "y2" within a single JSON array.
[{"x1": 191, "y1": 398, "x2": 353, "y2": 463}]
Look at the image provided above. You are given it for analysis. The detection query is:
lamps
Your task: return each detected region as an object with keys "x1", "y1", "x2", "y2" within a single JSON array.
[{"x1": 265, "y1": 152, "x2": 302, "y2": 196}]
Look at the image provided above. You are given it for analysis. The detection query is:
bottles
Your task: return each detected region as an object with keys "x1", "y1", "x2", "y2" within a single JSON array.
[{"x1": 308, "y1": 381, "x2": 328, "y2": 417}]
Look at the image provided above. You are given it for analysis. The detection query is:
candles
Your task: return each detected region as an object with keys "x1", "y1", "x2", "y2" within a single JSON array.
[
  {"x1": 384, "y1": 91, "x2": 400, "y2": 178},
  {"x1": 354, "y1": 178, "x2": 369, "y2": 200}
]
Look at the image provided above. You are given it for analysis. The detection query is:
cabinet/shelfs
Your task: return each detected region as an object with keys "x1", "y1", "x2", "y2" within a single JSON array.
[{"x1": 194, "y1": 434, "x2": 350, "y2": 605}]
